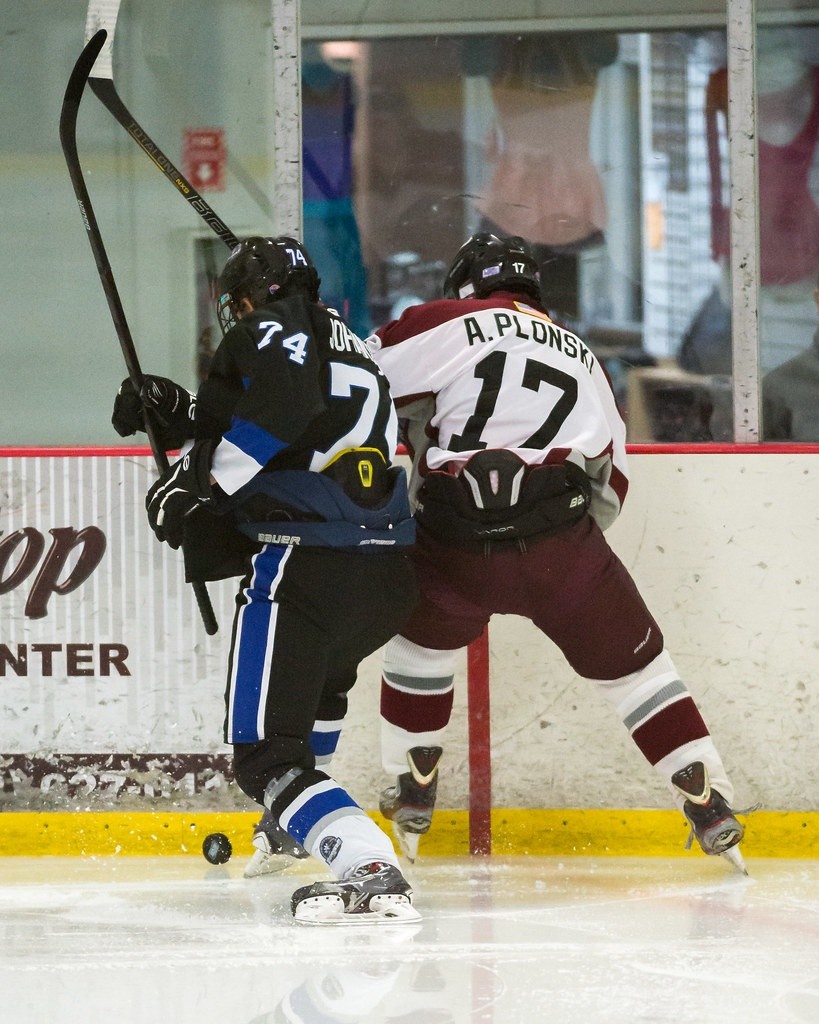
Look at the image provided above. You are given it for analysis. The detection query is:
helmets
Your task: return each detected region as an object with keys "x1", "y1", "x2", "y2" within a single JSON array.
[
  {"x1": 443, "y1": 231, "x2": 541, "y2": 300},
  {"x1": 215, "y1": 236, "x2": 319, "y2": 336}
]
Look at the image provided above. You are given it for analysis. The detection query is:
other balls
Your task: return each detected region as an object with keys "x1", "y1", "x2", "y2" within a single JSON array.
[{"x1": 202, "y1": 832, "x2": 232, "y2": 867}]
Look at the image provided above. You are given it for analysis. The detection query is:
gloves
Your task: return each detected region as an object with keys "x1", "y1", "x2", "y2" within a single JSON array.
[
  {"x1": 145, "y1": 439, "x2": 214, "y2": 550},
  {"x1": 113, "y1": 374, "x2": 199, "y2": 439}
]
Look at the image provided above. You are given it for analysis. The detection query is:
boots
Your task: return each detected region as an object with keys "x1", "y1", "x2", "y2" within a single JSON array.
[
  {"x1": 379, "y1": 746, "x2": 443, "y2": 864},
  {"x1": 670, "y1": 761, "x2": 749, "y2": 876}
]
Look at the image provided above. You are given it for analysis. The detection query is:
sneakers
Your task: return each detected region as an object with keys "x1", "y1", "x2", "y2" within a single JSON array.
[
  {"x1": 290, "y1": 862, "x2": 422, "y2": 925},
  {"x1": 242, "y1": 808, "x2": 310, "y2": 878}
]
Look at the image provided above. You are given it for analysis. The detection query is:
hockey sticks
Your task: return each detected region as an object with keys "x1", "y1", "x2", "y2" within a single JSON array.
[
  {"x1": 58, "y1": 30, "x2": 220, "y2": 635},
  {"x1": 82, "y1": 0, "x2": 415, "y2": 464}
]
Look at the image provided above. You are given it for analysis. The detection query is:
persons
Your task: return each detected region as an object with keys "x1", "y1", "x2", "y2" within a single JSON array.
[
  {"x1": 361, "y1": 233, "x2": 743, "y2": 856},
  {"x1": 298, "y1": 34, "x2": 621, "y2": 333},
  {"x1": 113, "y1": 238, "x2": 424, "y2": 923}
]
